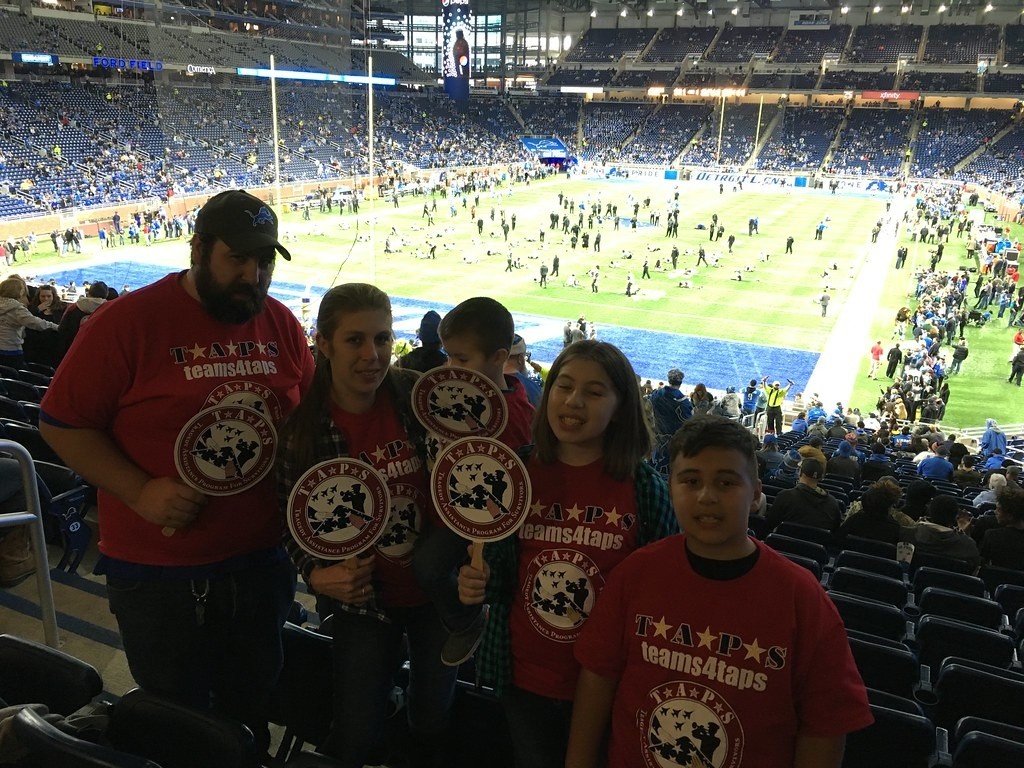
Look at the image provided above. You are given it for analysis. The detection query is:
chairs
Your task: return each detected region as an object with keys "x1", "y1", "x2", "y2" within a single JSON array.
[
  {"x1": 918, "y1": 589, "x2": 1003, "y2": 629},
  {"x1": 830, "y1": 566, "x2": 908, "y2": 610},
  {"x1": 747, "y1": 418, "x2": 1024, "y2": 540},
  {"x1": 835, "y1": 551, "x2": 904, "y2": 582},
  {"x1": 0, "y1": 0, "x2": 1024, "y2": 221},
  {"x1": 842, "y1": 688, "x2": 936, "y2": 768},
  {"x1": 0, "y1": 360, "x2": 513, "y2": 768},
  {"x1": 824, "y1": 590, "x2": 908, "y2": 641},
  {"x1": 932, "y1": 656, "x2": 1024, "y2": 731},
  {"x1": 844, "y1": 628, "x2": 918, "y2": 699},
  {"x1": 978, "y1": 566, "x2": 1024, "y2": 618},
  {"x1": 916, "y1": 613, "x2": 1015, "y2": 669},
  {"x1": 778, "y1": 550, "x2": 823, "y2": 583},
  {"x1": 950, "y1": 715, "x2": 1024, "y2": 768},
  {"x1": 773, "y1": 523, "x2": 833, "y2": 557},
  {"x1": 835, "y1": 536, "x2": 897, "y2": 560},
  {"x1": 909, "y1": 553, "x2": 974, "y2": 583},
  {"x1": 765, "y1": 533, "x2": 830, "y2": 567},
  {"x1": 912, "y1": 567, "x2": 985, "y2": 604}
]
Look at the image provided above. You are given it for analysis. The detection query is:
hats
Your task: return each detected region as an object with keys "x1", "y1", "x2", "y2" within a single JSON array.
[
  {"x1": 417, "y1": 310, "x2": 441, "y2": 343},
  {"x1": 838, "y1": 433, "x2": 857, "y2": 457},
  {"x1": 195, "y1": 189, "x2": 291, "y2": 263},
  {"x1": 801, "y1": 458, "x2": 824, "y2": 479}
]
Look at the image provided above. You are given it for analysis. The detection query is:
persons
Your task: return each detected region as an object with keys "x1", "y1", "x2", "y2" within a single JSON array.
[
  {"x1": 39, "y1": 190, "x2": 316, "y2": 768},
  {"x1": 566, "y1": 416, "x2": 872, "y2": 768},
  {"x1": 0, "y1": 0, "x2": 1024, "y2": 339},
  {"x1": 1, "y1": 275, "x2": 129, "y2": 372},
  {"x1": 441, "y1": 340, "x2": 679, "y2": 768},
  {"x1": 392, "y1": 304, "x2": 1024, "y2": 557},
  {"x1": 271, "y1": 284, "x2": 433, "y2": 768},
  {"x1": 400, "y1": 297, "x2": 535, "y2": 768}
]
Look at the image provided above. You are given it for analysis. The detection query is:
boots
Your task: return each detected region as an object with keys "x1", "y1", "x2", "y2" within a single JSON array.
[{"x1": 0, "y1": 525, "x2": 37, "y2": 587}]
